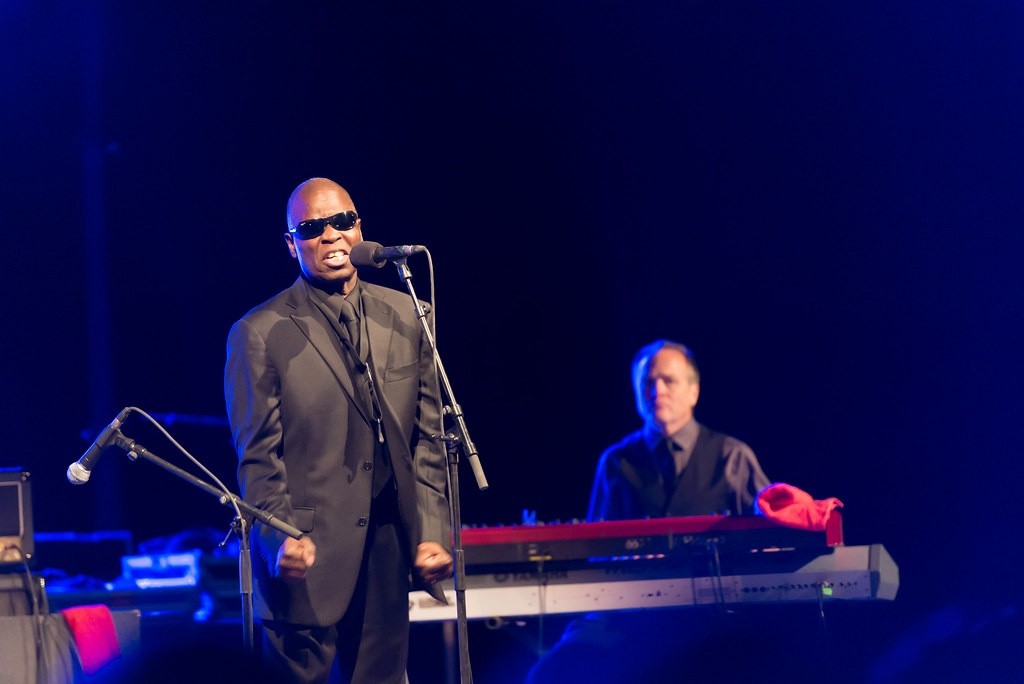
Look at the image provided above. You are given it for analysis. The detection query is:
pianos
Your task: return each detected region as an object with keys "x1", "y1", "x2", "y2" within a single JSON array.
[{"x1": 403, "y1": 543, "x2": 901, "y2": 623}]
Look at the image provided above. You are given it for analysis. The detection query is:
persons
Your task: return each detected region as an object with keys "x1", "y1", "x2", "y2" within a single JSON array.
[
  {"x1": 525, "y1": 340, "x2": 773, "y2": 684},
  {"x1": 224, "y1": 177, "x2": 455, "y2": 684}
]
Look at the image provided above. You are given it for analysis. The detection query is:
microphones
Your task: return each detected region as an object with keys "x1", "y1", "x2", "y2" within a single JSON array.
[
  {"x1": 350, "y1": 242, "x2": 426, "y2": 269},
  {"x1": 67, "y1": 407, "x2": 132, "y2": 485}
]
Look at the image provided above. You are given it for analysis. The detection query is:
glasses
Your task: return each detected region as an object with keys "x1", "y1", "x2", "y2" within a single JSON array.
[{"x1": 289, "y1": 211, "x2": 358, "y2": 240}]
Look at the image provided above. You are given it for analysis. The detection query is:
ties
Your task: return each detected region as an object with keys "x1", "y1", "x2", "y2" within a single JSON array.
[
  {"x1": 342, "y1": 299, "x2": 362, "y2": 354},
  {"x1": 661, "y1": 437, "x2": 677, "y2": 511}
]
